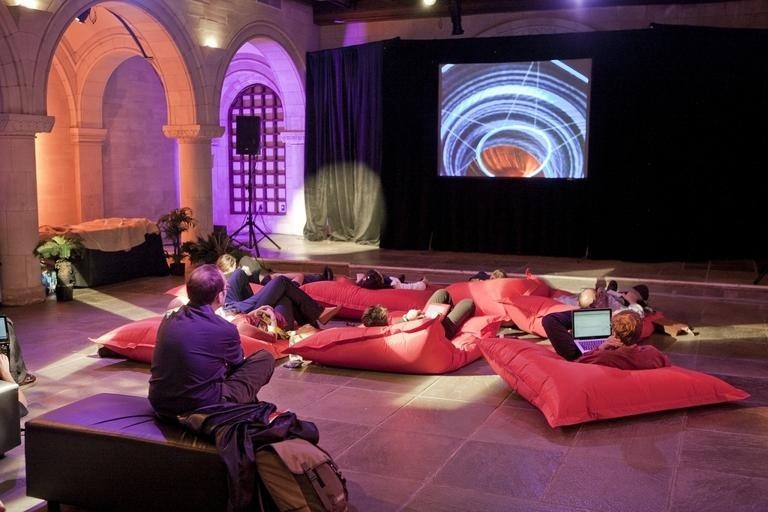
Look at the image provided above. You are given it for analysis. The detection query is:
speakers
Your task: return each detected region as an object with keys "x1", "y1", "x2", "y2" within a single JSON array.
[{"x1": 236, "y1": 115, "x2": 260, "y2": 155}]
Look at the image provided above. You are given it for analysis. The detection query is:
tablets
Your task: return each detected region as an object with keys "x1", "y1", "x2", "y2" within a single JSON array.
[{"x1": 420, "y1": 300, "x2": 451, "y2": 324}]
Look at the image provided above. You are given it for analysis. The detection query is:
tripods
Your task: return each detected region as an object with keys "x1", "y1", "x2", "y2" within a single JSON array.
[{"x1": 223, "y1": 157, "x2": 282, "y2": 255}]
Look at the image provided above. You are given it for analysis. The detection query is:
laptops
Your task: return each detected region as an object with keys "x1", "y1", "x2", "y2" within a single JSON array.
[
  {"x1": 572, "y1": 308, "x2": 612, "y2": 355},
  {"x1": 0, "y1": 316, "x2": 11, "y2": 359}
]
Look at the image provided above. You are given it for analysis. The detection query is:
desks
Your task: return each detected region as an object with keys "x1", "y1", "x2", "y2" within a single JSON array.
[{"x1": 38, "y1": 217, "x2": 170, "y2": 287}]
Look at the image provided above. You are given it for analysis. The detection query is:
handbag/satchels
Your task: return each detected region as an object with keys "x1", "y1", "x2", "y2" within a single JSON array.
[{"x1": 254, "y1": 437, "x2": 348, "y2": 512}]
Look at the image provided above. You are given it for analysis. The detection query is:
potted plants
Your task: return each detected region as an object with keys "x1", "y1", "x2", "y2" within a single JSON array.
[
  {"x1": 156, "y1": 207, "x2": 199, "y2": 275},
  {"x1": 34, "y1": 235, "x2": 86, "y2": 302}
]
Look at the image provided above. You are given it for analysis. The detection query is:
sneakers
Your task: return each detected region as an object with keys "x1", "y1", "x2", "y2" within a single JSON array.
[
  {"x1": 18, "y1": 373, "x2": 36, "y2": 386},
  {"x1": 322, "y1": 264, "x2": 332, "y2": 281},
  {"x1": 319, "y1": 304, "x2": 343, "y2": 325}
]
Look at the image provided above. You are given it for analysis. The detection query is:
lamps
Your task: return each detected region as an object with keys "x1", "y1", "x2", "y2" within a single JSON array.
[{"x1": 75, "y1": 8, "x2": 91, "y2": 24}]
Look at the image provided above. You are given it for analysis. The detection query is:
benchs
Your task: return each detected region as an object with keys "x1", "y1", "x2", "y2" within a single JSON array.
[{"x1": 25, "y1": 394, "x2": 228, "y2": 512}]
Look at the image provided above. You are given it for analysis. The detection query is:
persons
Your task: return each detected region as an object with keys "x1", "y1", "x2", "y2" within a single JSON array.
[
  {"x1": 0, "y1": 352, "x2": 30, "y2": 420},
  {"x1": 148, "y1": 263, "x2": 278, "y2": 423},
  {"x1": 212, "y1": 253, "x2": 654, "y2": 363},
  {"x1": 0, "y1": 312, "x2": 36, "y2": 388}
]
